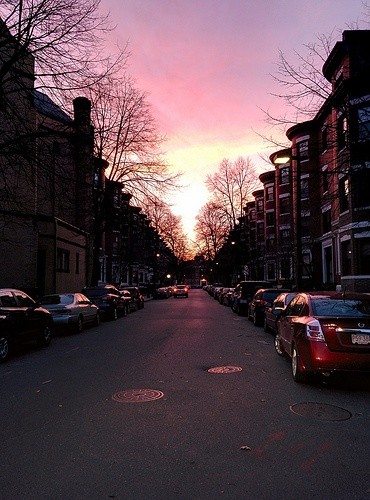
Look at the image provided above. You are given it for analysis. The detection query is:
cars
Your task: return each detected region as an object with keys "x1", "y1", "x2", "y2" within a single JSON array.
[
  {"x1": 34, "y1": 292, "x2": 102, "y2": 335},
  {"x1": 263, "y1": 292, "x2": 297, "y2": 333},
  {"x1": 248, "y1": 288, "x2": 291, "y2": 328},
  {"x1": 138, "y1": 281, "x2": 202, "y2": 300},
  {"x1": 274, "y1": 290, "x2": 370, "y2": 385},
  {"x1": 119, "y1": 285, "x2": 145, "y2": 315},
  {"x1": 203, "y1": 284, "x2": 236, "y2": 307}
]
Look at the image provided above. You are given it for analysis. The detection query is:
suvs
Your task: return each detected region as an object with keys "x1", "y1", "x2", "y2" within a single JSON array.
[
  {"x1": 231, "y1": 280, "x2": 275, "y2": 316},
  {"x1": 80, "y1": 283, "x2": 128, "y2": 321},
  {"x1": 0, "y1": 288, "x2": 55, "y2": 365}
]
